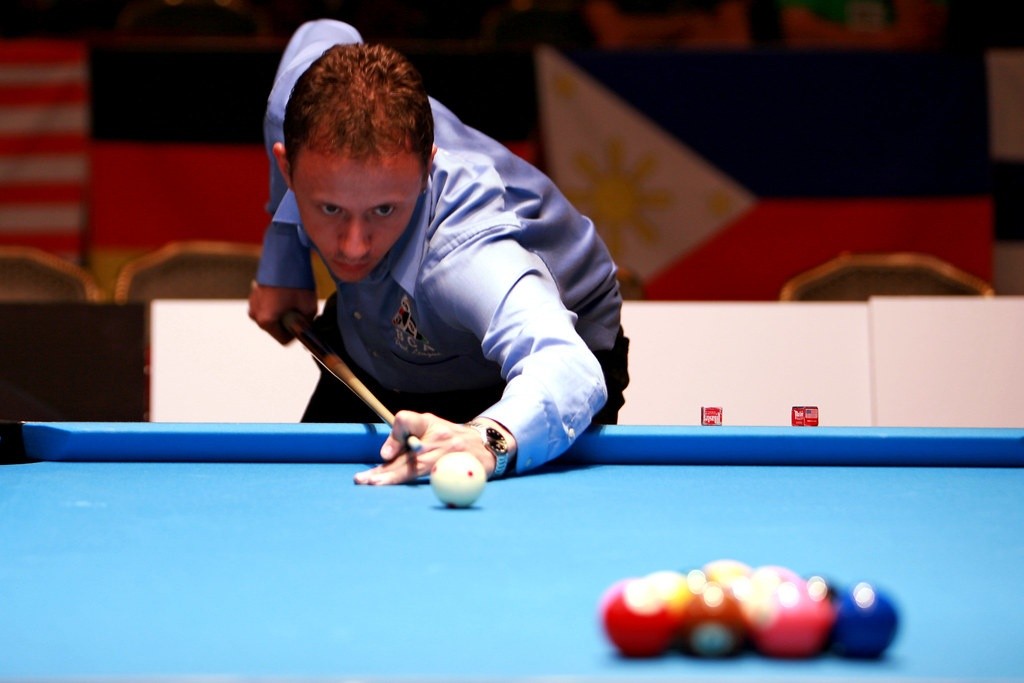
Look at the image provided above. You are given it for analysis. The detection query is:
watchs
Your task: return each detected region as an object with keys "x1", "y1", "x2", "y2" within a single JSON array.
[{"x1": 468, "y1": 422, "x2": 508, "y2": 478}]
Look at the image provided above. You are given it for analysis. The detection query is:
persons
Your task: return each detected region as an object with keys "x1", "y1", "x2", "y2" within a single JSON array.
[{"x1": 249, "y1": 19, "x2": 630, "y2": 489}]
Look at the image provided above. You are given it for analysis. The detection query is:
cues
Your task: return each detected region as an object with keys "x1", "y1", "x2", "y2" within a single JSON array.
[{"x1": 249, "y1": 278, "x2": 423, "y2": 454}]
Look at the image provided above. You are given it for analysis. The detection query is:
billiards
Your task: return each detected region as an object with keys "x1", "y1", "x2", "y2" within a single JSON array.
[
  {"x1": 600, "y1": 557, "x2": 898, "y2": 660},
  {"x1": 428, "y1": 451, "x2": 488, "y2": 508}
]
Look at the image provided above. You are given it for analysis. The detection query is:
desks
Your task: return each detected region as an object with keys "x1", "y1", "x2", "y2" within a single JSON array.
[
  {"x1": 0, "y1": 421, "x2": 1024, "y2": 683},
  {"x1": 0, "y1": 292, "x2": 1024, "y2": 428}
]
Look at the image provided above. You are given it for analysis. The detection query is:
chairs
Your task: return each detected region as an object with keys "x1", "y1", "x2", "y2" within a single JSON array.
[
  {"x1": 114, "y1": 237, "x2": 261, "y2": 299},
  {"x1": 778, "y1": 252, "x2": 997, "y2": 303}
]
